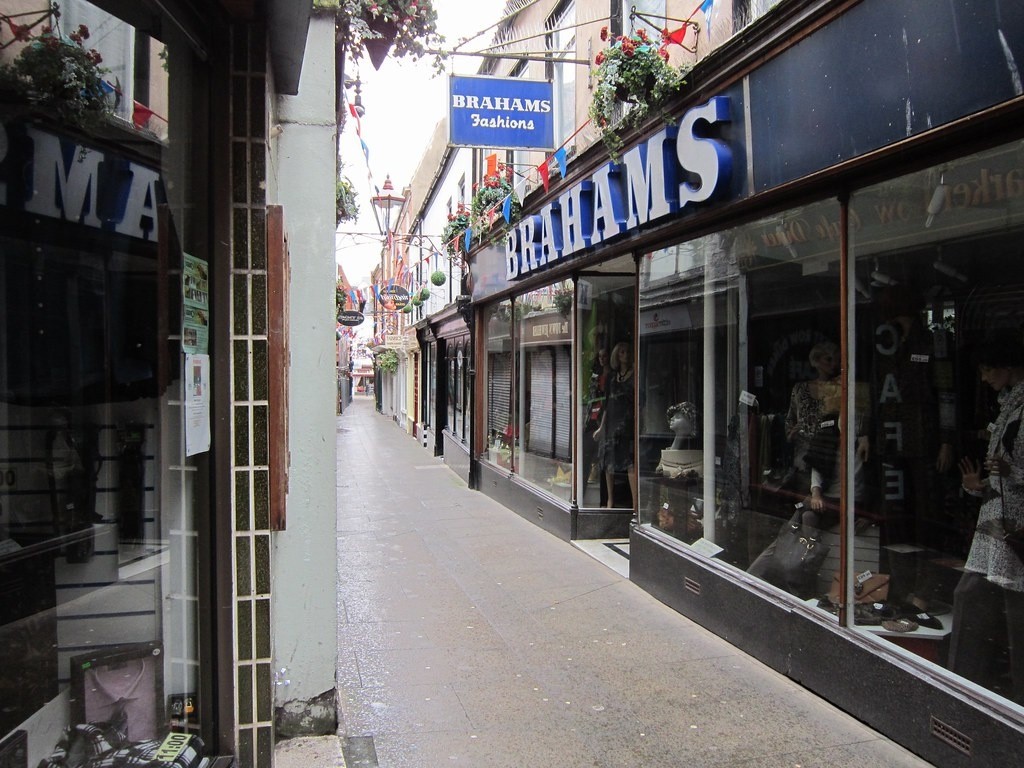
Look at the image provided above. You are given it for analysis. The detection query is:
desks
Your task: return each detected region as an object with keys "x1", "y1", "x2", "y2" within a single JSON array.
[{"x1": 647, "y1": 476, "x2": 738, "y2": 565}]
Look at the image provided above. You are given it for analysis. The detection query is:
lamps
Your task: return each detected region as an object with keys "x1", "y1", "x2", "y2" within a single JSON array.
[
  {"x1": 925, "y1": 166, "x2": 955, "y2": 230},
  {"x1": 775, "y1": 217, "x2": 800, "y2": 260},
  {"x1": 932, "y1": 245, "x2": 971, "y2": 287},
  {"x1": 855, "y1": 256, "x2": 898, "y2": 297}
]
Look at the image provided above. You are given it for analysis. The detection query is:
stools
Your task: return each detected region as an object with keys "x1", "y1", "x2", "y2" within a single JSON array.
[{"x1": 820, "y1": 522, "x2": 884, "y2": 595}]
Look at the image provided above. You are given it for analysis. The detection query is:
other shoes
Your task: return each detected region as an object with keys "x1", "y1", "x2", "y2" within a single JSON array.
[{"x1": 902, "y1": 603, "x2": 943, "y2": 629}]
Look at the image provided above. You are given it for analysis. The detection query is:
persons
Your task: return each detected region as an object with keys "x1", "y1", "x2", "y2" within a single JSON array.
[
  {"x1": 592, "y1": 342, "x2": 636, "y2": 509},
  {"x1": 945, "y1": 339, "x2": 1024, "y2": 706},
  {"x1": 666, "y1": 400, "x2": 702, "y2": 437},
  {"x1": 769, "y1": 402, "x2": 882, "y2": 597},
  {"x1": 783, "y1": 340, "x2": 843, "y2": 513},
  {"x1": 581, "y1": 343, "x2": 613, "y2": 508}
]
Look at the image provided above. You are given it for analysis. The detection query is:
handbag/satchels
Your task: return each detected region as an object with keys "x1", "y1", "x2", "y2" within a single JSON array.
[
  {"x1": 1004, "y1": 527, "x2": 1024, "y2": 564},
  {"x1": 774, "y1": 506, "x2": 828, "y2": 589},
  {"x1": 829, "y1": 570, "x2": 891, "y2": 605}
]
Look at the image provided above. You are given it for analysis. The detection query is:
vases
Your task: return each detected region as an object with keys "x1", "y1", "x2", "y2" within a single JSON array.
[{"x1": 609, "y1": 71, "x2": 657, "y2": 104}]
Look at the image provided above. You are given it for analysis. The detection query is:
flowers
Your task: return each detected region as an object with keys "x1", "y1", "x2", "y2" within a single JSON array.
[
  {"x1": 441, "y1": 203, "x2": 470, "y2": 254},
  {"x1": 472, "y1": 164, "x2": 521, "y2": 247},
  {"x1": 336, "y1": 0, "x2": 450, "y2": 77},
  {"x1": 589, "y1": 25, "x2": 687, "y2": 165},
  {"x1": 3, "y1": 24, "x2": 115, "y2": 132}
]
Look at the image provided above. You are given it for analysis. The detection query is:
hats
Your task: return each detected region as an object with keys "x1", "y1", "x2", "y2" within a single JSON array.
[{"x1": 667, "y1": 402, "x2": 701, "y2": 439}]
